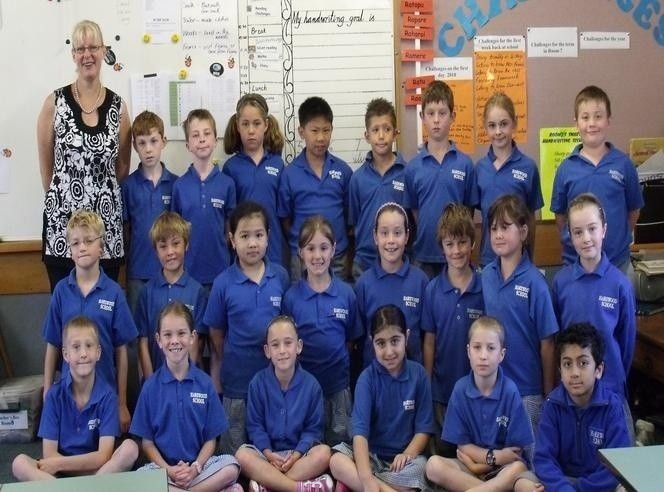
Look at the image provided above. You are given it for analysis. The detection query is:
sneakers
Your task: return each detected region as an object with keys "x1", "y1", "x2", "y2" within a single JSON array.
[
  {"x1": 297, "y1": 473, "x2": 335, "y2": 492},
  {"x1": 248, "y1": 479, "x2": 269, "y2": 491},
  {"x1": 221, "y1": 483, "x2": 245, "y2": 492}
]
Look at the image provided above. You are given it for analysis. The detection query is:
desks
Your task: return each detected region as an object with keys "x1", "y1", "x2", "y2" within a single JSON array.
[
  {"x1": 597, "y1": 444, "x2": 664, "y2": 492},
  {"x1": 1, "y1": 468, "x2": 168, "y2": 491}
]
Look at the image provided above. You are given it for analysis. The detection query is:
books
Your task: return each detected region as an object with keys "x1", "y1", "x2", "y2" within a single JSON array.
[{"x1": 637, "y1": 259, "x2": 664, "y2": 276}]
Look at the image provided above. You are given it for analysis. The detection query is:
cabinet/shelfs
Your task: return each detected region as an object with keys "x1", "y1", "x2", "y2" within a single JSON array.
[{"x1": 634, "y1": 310, "x2": 663, "y2": 380}]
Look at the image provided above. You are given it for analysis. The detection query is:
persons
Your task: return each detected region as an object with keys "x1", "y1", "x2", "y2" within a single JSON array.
[{"x1": 14, "y1": 17, "x2": 646, "y2": 492}]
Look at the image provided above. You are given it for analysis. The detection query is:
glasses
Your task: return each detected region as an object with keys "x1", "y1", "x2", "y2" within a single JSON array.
[
  {"x1": 73, "y1": 44, "x2": 104, "y2": 54},
  {"x1": 65, "y1": 233, "x2": 104, "y2": 247}
]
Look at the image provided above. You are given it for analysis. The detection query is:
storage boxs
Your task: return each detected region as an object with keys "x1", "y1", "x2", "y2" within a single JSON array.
[{"x1": 0, "y1": 376, "x2": 45, "y2": 443}]
